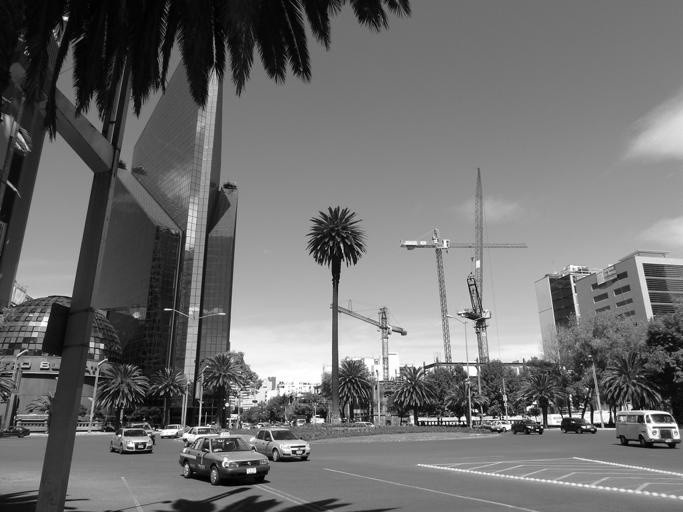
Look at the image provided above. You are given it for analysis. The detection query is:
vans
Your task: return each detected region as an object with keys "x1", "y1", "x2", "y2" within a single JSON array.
[{"x1": 616, "y1": 410, "x2": 681, "y2": 448}]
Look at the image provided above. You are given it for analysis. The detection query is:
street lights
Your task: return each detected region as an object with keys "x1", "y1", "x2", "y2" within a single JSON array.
[
  {"x1": 465, "y1": 378, "x2": 472, "y2": 429},
  {"x1": 88, "y1": 358, "x2": 108, "y2": 433},
  {"x1": 164, "y1": 308, "x2": 226, "y2": 426},
  {"x1": 588, "y1": 355, "x2": 605, "y2": 428},
  {"x1": 199, "y1": 366, "x2": 211, "y2": 426},
  {"x1": 1, "y1": 349, "x2": 29, "y2": 433}
]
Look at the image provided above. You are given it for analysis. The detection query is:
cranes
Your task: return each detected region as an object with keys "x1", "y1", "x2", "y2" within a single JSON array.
[
  {"x1": 459, "y1": 166, "x2": 491, "y2": 363},
  {"x1": 400, "y1": 228, "x2": 528, "y2": 369},
  {"x1": 330, "y1": 300, "x2": 407, "y2": 381}
]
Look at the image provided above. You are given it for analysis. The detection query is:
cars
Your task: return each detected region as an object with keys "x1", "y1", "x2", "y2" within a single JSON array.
[
  {"x1": 249, "y1": 429, "x2": 311, "y2": 462},
  {"x1": 182, "y1": 426, "x2": 221, "y2": 447},
  {"x1": 179, "y1": 437, "x2": 270, "y2": 485},
  {"x1": 0, "y1": 426, "x2": 30, "y2": 438},
  {"x1": 483, "y1": 420, "x2": 544, "y2": 435},
  {"x1": 355, "y1": 421, "x2": 375, "y2": 428},
  {"x1": 561, "y1": 418, "x2": 597, "y2": 434},
  {"x1": 160, "y1": 424, "x2": 183, "y2": 438},
  {"x1": 110, "y1": 422, "x2": 155, "y2": 454},
  {"x1": 241, "y1": 415, "x2": 325, "y2": 430}
]
